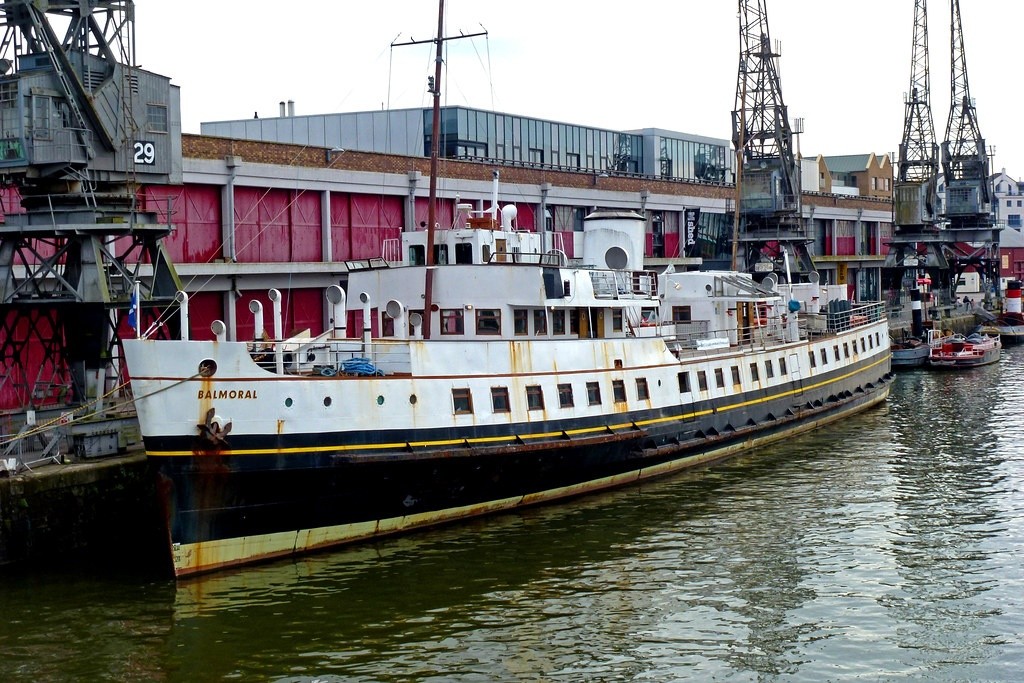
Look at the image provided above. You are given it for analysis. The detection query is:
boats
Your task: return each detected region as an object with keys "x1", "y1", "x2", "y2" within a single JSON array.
[
  {"x1": 122, "y1": 0, "x2": 896, "y2": 576},
  {"x1": 891, "y1": 312, "x2": 1024, "y2": 367}
]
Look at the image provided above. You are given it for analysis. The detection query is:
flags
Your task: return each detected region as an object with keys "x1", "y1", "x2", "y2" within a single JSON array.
[{"x1": 128, "y1": 286, "x2": 137, "y2": 332}]
